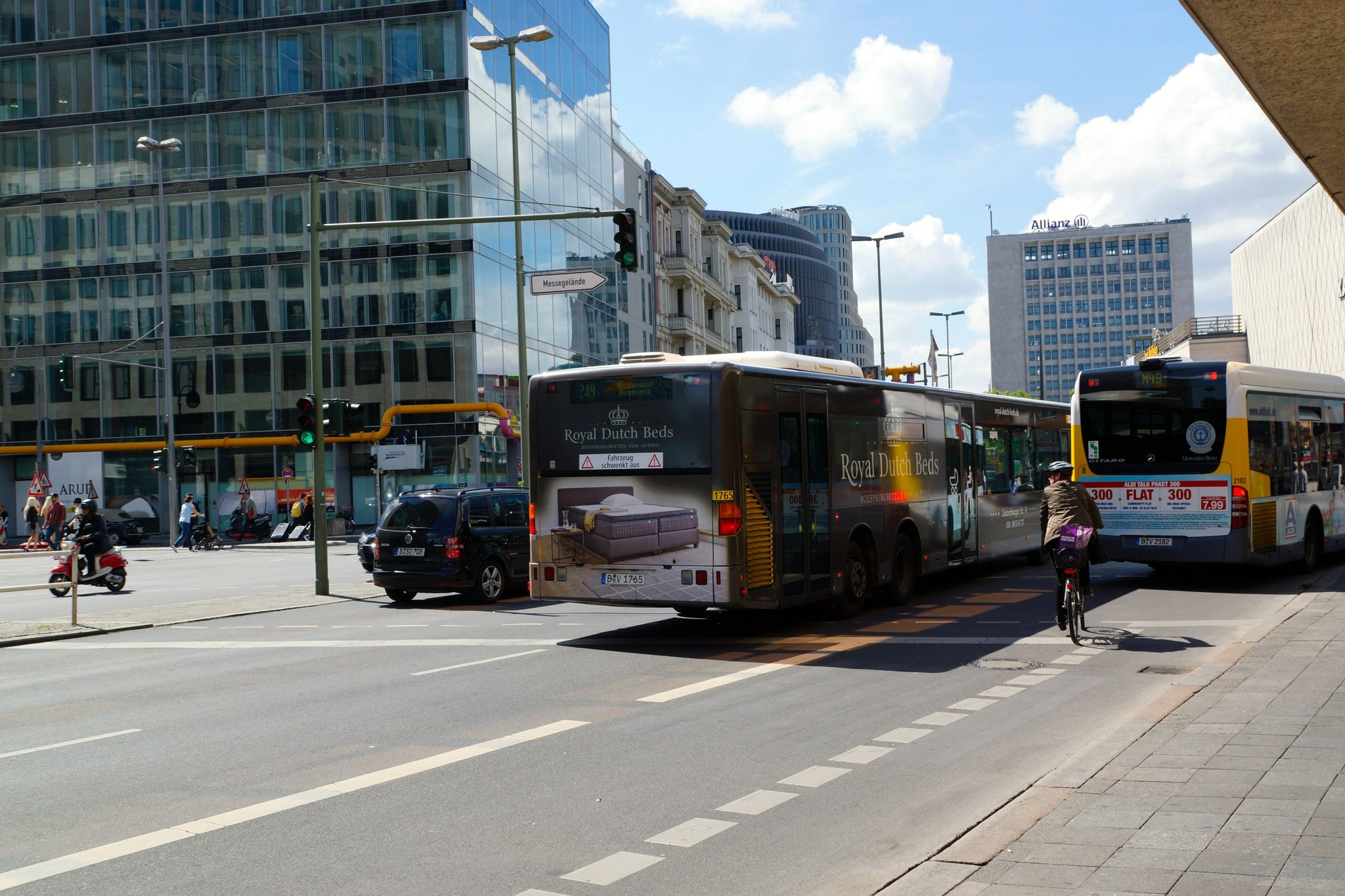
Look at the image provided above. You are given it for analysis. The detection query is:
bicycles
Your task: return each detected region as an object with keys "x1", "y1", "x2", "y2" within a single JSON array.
[
  {"x1": 334, "y1": 512, "x2": 356, "y2": 535},
  {"x1": 1040, "y1": 522, "x2": 1096, "y2": 643}
]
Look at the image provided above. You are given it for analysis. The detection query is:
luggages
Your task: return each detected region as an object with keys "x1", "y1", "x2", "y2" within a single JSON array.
[
  {"x1": 271, "y1": 520, "x2": 294, "y2": 542},
  {"x1": 288, "y1": 523, "x2": 311, "y2": 541}
]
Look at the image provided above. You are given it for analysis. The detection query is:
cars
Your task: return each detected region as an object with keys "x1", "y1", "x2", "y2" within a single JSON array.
[
  {"x1": 356, "y1": 524, "x2": 379, "y2": 572},
  {"x1": 61, "y1": 508, "x2": 151, "y2": 546}
]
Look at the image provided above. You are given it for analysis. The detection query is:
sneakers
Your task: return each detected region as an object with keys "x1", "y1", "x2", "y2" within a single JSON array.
[
  {"x1": 54, "y1": 556, "x2": 60, "y2": 560},
  {"x1": 83, "y1": 572, "x2": 97, "y2": 578}
]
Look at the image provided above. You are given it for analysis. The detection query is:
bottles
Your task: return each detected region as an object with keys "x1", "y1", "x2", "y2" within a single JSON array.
[{"x1": 562, "y1": 510, "x2": 569, "y2": 528}]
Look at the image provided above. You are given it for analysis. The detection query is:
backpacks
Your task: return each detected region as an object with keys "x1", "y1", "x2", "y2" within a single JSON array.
[{"x1": 291, "y1": 500, "x2": 305, "y2": 518}]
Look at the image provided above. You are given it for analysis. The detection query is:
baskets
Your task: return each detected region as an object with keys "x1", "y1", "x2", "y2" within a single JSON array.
[{"x1": 1053, "y1": 549, "x2": 1087, "y2": 569}]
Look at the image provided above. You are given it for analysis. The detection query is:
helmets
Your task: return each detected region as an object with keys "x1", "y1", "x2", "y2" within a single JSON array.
[
  {"x1": 1044, "y1": 461, "x2": 1074, "y2": 471},
  {"x1": 78, "y1": 499, "x2": 97, "y2": 516},
  {"x1": 75, "y1": 507, "x2": 83, "y2": 522}
]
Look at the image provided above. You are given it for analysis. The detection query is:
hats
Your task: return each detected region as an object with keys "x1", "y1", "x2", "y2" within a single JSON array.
[
  {"x1": 49, "y1": 493, "x2": 58, "y2": 497},
  {"x1": 73, "y1": 498, "x2": 81, "y2": 503},
  {"x1": 184, "y1": 493, "x2": 193, "y2": 497}
]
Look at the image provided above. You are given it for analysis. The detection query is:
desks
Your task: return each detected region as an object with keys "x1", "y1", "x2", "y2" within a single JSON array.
[{"x1": 550, "y1": 526, "x2": 585, "y2": 568}]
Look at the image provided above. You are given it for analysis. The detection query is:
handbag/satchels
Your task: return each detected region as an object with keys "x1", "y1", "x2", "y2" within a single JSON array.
[{"x1": 1060, "y1": 525, "x2": 1093, "y2": 548}]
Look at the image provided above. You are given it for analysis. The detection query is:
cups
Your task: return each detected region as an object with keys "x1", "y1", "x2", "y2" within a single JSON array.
[{"x1": 571, "y1": 523, "x2": 577, "y2": 531}]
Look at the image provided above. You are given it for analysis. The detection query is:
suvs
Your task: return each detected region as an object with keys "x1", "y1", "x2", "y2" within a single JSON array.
[{"x1": 370, "y1": 484, "x2": 530, "y2": 605}]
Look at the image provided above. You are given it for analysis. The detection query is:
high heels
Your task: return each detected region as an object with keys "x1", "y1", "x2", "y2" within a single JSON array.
[{"x1": 23, "y1": 548, "x2": 29, "y2": 553}]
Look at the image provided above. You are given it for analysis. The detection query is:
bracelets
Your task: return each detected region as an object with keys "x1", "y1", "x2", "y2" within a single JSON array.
[{"x1": 59, "y1": 525, "x2": 63, "y2": 527}]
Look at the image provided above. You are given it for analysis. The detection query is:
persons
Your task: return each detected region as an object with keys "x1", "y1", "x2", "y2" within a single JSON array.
[
  {"x1": 0, "y1": 506, "x2": 8, "y2": 539},
  {"x1": 1323, "y1": 491, "x2": 1341, "y2": 536},
  {"x1": 1040, "y1": 462, "x2": 1103, "y2": 631},
  {"x1": 68, "y1": 498, "x2": 113, "y2": 578},
  {"x1": 291, "y1": 493, "x2": 314, "y2": 540},
  {"x1": 234, "y1": 493, "x2": 263, "y2": 542},
  {"x1": 1292, "y1": 462, "x2": 1308, "y2": 494},
  {"x1": 207, "y1": 523, "x2": 222, "y2": 541},
  {"x1": 172, "y1": 493, "x2": 205, "y2": 553},
  {"x1": 23, "y1": 493, "x2": 84, "y2": 552}
]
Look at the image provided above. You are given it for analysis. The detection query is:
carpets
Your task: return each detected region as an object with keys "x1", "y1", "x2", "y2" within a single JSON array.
[{"x1": 583, "y1": 565, "x2": 728, "y2": 602}]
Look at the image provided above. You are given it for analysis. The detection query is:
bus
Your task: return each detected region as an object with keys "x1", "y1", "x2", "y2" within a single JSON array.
[
  {"x1": 521, "y1": 347, "x2": 1074, "y2": 625},
  {"x1": 1066, "y1": 354, "x2": 1345, "y2": 583}
]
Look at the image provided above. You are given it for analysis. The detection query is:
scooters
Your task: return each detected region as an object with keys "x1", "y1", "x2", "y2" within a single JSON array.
[
  {"x1": 225, "y1": 506, "x2": 273, "y2": 539},
  {"x1": 48, "y1": 524, "x2": 128, "y2": 597}
]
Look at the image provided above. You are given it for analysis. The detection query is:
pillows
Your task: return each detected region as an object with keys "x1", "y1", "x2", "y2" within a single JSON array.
[{"x1": 600, "y1": 493, "x2": 644, "y2": 506}]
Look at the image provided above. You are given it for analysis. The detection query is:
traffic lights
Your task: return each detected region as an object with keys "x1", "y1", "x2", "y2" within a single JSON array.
[
  {"x1": 293, "y1": 396, "x2": 317, "y2": 451},
  {"x1": 328, "y1": 401, "x2": 364, "y2": 436},
  {"x1": 177, "y1": 445, "x2": 196, "y2": 467},
  {"x1": 59, "y1": 353, "x2": 75, "y2": 392},
  {"x1": 153, "y1": 448, "x2": 168, "y2": 474},
  {"x1": 611, "y1": 209, "x2": 640, "y2": 270}
]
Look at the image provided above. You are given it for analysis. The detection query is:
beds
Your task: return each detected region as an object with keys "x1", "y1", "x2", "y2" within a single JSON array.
[{"x1": 557, "y1": 486, "x2": 699, "y2": 563}]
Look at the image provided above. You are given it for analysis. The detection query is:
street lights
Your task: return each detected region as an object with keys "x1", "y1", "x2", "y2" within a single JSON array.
[
  {"x1": 929, "y1": 310, "x2": 965, "y2": 389},
  {"x1": 468, "y1": 22, "x2": 555, "y2": 491},
  {"x1": 926, "y1": 374, "x2": 948, "y2": 387},
  {"x1": 133, "y1": 136, "x2": 182, "y2": 552},
  {"x1": 937, "y1": 352, "x2": 964, "y2": 389},
  {"x1": 851, "y1": 231, "x2": 905, "y2": 382}
]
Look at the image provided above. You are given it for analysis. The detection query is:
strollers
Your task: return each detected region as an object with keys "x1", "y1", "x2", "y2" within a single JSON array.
[{"x1": 190, "y1": 517, "x2": 224, "y2": 551}]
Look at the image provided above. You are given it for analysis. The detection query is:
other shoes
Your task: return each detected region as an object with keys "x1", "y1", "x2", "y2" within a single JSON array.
[
  {"x1": 1084, "y1": 586, "x2": 1093, "y2": 597},
  {"x1": 1055, "y1": 615, "x2": 1067, "y2": 631},
  {"x1": 190, "y1": 548, "x2": 196, "y2": 552},
  {"x1": 256, "y1": 538, "x2": 263, "y2": 542},
  {"x1": 235, "y1": 537, "x2": 243, "y2": 542},
  {"x1": 172, "y1": 545, "x2": 178, "y2": 553}
]
